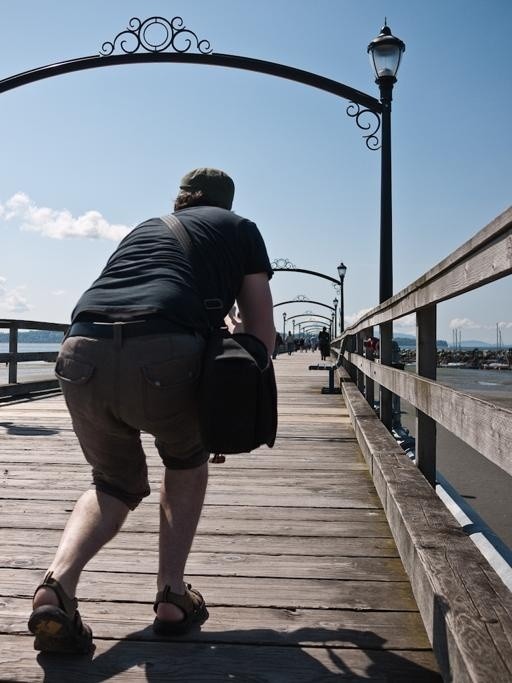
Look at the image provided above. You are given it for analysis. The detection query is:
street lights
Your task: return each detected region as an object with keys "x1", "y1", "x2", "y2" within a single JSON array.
[
  {"x1": 280, "y1": 314, "x2": 308, "y2": 340},
  {"x1": 330, "y1": 262, "x2": 348, "y2": 338},
  {"x1": 369, "y1": 17, "x2": 406, "y2": 433}
]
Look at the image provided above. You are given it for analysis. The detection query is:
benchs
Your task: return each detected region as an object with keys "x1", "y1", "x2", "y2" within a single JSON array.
[{"x1": 309, "y1": 338, "x2": 347, "y2": 394}]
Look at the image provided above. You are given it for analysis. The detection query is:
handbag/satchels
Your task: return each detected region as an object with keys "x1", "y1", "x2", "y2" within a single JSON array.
[{"x1": 196, "y1": 334, "x2": 277, "y2": 455}]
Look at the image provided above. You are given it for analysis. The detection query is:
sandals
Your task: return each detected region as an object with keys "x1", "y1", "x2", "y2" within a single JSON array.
[
  {"x1": 152, "y1": 582, "x2": 206, "y2": 636},
  {"x1": 28, "y1": 572, "x2": 91, "y2": 652}
]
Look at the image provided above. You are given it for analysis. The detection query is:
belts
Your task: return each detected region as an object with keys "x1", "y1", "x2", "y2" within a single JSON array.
[{"x1": 68, "y1": 319, "x2": 190, "y2": 338}]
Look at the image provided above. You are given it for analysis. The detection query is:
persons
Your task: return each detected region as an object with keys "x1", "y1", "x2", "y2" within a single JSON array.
[
  {"x1": 272, "y1": 326, "x2": 331, "y2": 361},
  {"x1": 28, "y1": 168, "x2": 277, "y2": 653}
]
